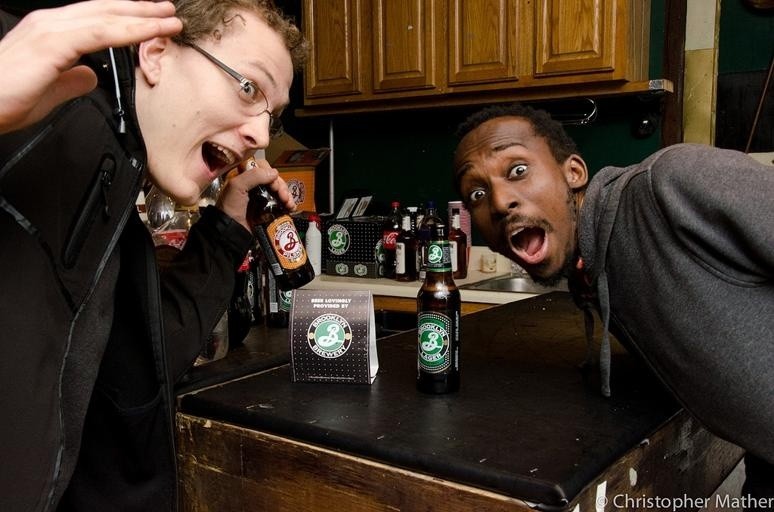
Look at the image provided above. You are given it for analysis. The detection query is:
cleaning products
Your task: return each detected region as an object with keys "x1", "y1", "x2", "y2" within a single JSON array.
[{"x1": 304, "y1": 212, "x2": 325, "y2": 276}]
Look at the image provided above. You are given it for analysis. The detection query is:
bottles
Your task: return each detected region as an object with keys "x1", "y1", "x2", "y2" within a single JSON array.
[
  {"x1": 395, "y1": 215, "x2": 416, "y2": 282},
  {"x1": 305, "y1": 214, "x2": 322, "y2": 276},
  {"x1": 416, "y1": 225, "x2": 461, "y2": 393},
  {"x1": 384, "y1": 201, "x2": 405, "y2": 279},
  {"x1": 135, "y1": 175, "x2": 294, "y2": 367},
  {"x1": 406, "y1": 200, "x2": 445, "y2": 283},
  {"x1": 234, "y1": 155, "x2": 317, "y2": 292},
  {"x1": 447, "y1": 206, "x2": 468, "y2": 279}
]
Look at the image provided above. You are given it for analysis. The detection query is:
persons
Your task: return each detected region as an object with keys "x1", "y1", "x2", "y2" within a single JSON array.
[
  {"x1": 0, "y1": 1, "x2": 300, "y2": 512},
  {"x1": 444, "y1": 95, "x2": 773, "y2": 512}
]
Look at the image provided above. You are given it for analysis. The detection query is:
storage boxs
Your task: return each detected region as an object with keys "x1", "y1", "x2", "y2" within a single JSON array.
[
  {"x1": 293, "y1": 214, "x2": 337, "y2": 272},
  {"x1": 265, "y1": 137, "x2": 331, "y2": 218},
  {"x1": 326, "y1": 215, "x2": 391, "y2": 278}
]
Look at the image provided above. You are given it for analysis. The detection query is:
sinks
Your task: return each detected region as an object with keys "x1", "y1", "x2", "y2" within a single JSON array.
[{"x1": 456, "y1": 270, "x2": 571, "y2": 295}]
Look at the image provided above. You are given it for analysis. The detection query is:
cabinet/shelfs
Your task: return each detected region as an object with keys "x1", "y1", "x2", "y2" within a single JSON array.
[
  {"x1": 301, "y1": 0, "x2": 444, "y2": 117},
  {"x1": 443, "y1": 0, "x2": 652, "y2": 107}
]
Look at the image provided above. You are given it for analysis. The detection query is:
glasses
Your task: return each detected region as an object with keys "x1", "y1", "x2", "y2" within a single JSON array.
[{"x1": 186, "y1": 41, "x2": 285, "y2": 138}]
